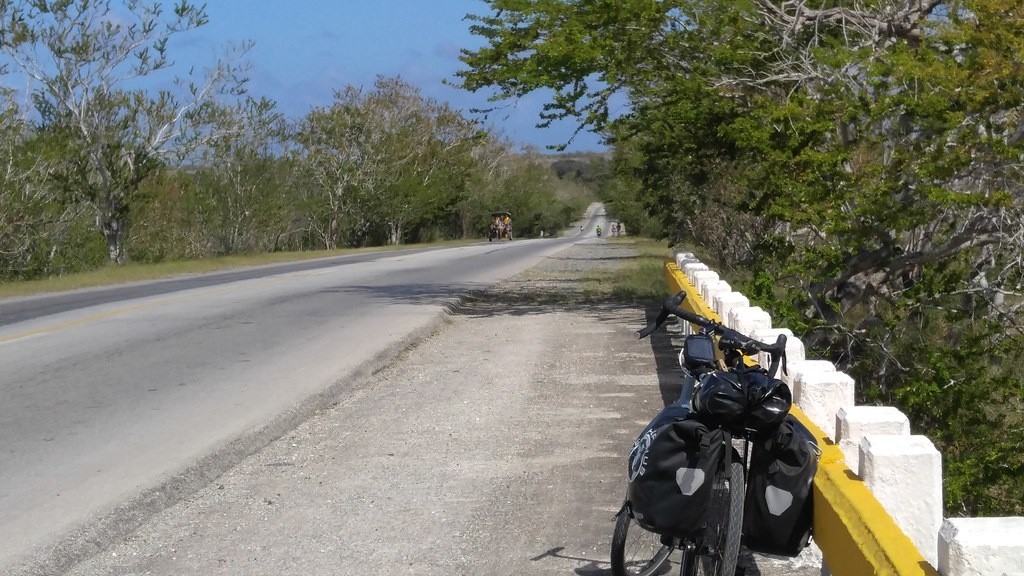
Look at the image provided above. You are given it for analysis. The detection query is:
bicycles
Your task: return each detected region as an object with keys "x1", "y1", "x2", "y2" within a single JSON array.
[{"x1": 610, "y1": 290, "x2": 788, "y2": 576}]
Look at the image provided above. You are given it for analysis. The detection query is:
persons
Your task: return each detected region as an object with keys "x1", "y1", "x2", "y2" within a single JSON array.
[
  {"x1": 612, "y1": 223, "x2": 621, "y2": 237},
  {"x1": 494, "y1": 215, "x2": 509, "y2": 225},
  {"x1": 596, "y1": 225, "x2": 601, "y2": 234}
]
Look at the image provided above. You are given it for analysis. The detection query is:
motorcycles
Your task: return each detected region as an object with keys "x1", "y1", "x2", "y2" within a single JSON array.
[{"x1": 488, "y1": 211, "x2": 513, "y2": 241}]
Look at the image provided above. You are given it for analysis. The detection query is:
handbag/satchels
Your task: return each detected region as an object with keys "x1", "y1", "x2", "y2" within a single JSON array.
[{"x1": 627, "y1": 369, "x2": 822, "y2": 558}]
